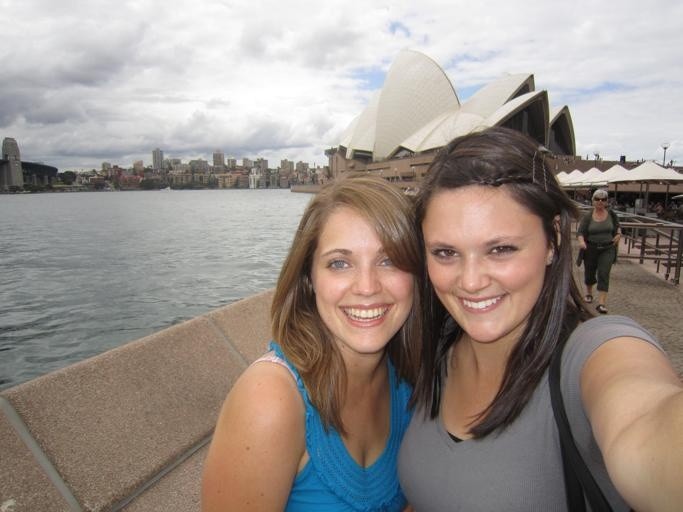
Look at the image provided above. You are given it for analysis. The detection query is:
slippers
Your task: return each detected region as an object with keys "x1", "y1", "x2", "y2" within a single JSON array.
[
  {"x1": 584, "y1": 295, "x2": 592, "y2": 303},
  {"x1": 596, "y1": 305, "x2": 608, "y2": 313}
]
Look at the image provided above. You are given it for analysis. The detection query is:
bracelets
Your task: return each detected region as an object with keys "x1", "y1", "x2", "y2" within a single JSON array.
[{"x1": 617, "y1": 231, "x2": 621, "y2": 237}]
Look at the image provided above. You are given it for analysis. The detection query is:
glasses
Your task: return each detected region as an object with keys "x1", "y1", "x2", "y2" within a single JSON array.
[{"x1": 595, "y1": 197, "x2": 607, "y2": 201}]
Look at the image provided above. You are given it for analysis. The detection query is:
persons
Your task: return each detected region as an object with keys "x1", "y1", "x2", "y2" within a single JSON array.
[
  {"x1": 605, "y1": 197, "x2": 683, "y2": 216},
  {"x1": 386, "y1": 124, "x2": 683, "y2": 512},
  {"x1": 575, "y1": 189, "x2": 622, "y2": 314},
  {"x1": 202, "y1": 174, "x2": 446, "y2": 511}
]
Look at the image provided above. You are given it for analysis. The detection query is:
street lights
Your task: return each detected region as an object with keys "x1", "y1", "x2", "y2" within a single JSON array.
[
  {"x1": 412, "y1": 167, "x2": 416, "y2": 183},
  {"x1": 394, "y1": 168, "x2": 398, "y2": 183},
  {"x1": 379, "y1": 170, "x2": 384, "y2": 181},
  {"x1": 660, "y1": 143, "x2": 669, "y2": 168}
]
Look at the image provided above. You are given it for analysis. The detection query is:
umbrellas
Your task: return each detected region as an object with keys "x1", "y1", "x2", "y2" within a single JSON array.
[{"x1": 555, "y1": 160, "x2": 683, "y2": 206}]
